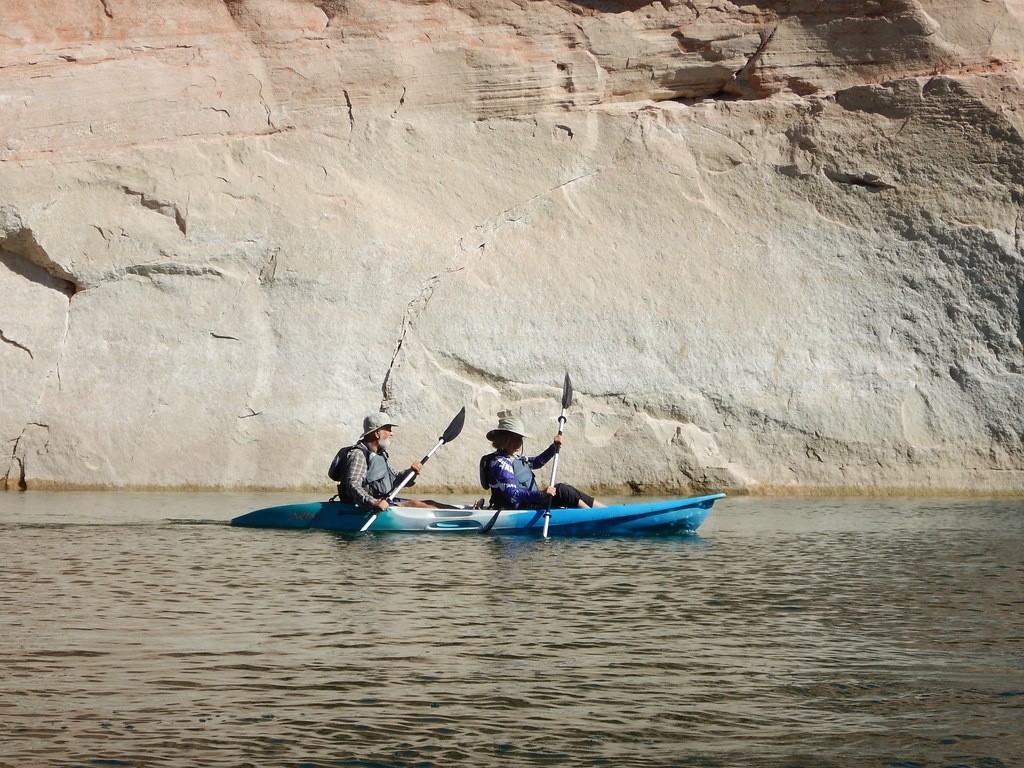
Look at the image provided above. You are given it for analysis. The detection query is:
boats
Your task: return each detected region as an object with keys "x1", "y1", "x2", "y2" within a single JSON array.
[{"x1": 228, "y1": 493, "x2": 730, "y2": 540}]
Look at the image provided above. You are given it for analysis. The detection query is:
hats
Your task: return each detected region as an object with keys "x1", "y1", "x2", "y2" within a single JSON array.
[
  {"x1": 486, "y1": 416, "x2": 530, "y2": 441},
  {"x1": 359, "y1": 412, "x2": 398, "y2": 437}
]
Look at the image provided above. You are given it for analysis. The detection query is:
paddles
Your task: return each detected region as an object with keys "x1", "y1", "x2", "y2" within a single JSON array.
[
  {"x1": 358, "y1": 405, "x2": 467, "y2": 532},
  {"x1": 541, "y1": 371, "x2": 575, "y2": 538}
]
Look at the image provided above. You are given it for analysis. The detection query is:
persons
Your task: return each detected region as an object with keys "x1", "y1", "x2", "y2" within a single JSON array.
[
  {"x1": 485, "y1": 416, "x2": 604, "y2": 510},
  {"x1": 341, "y1": 412, "x2": 485, "y2": 511}
]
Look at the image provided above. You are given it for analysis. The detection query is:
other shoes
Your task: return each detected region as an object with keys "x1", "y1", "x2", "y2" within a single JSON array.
[{"x1": 464, "y1": 498, "x2": 484, "y2": 510}]
